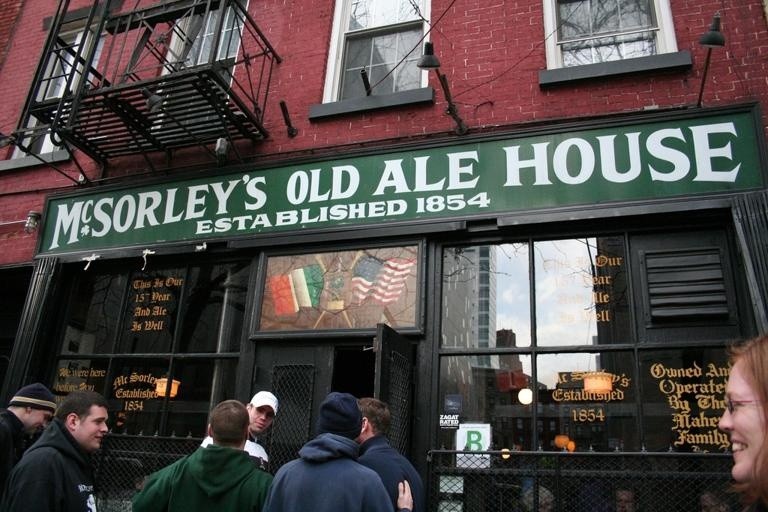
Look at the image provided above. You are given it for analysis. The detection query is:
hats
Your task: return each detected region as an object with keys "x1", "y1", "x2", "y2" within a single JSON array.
[
  {"x1": 317, "y1": 392, "x2": 362, "y2": 440},
  {"x1": 7, "y1": 383, "x2": 58, "y2": 415},
  {"x1": 250, "y1": 391, "x2": 279, "y2": 417}
]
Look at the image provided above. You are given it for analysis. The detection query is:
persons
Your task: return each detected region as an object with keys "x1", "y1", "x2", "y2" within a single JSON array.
[
  {"x1": 198, "y1": 389, "x2": 279, "y2": 467},
  {"x1": 1, "y1": 382, "x2": 56, "y2": 512},
  {"x1": 718, "y1": 332, "x2": 767, "y2": 512},
  {"x1": 130, "y1": 398, "x2": 273, "y2": 511},
  {"x1": 523, "y1": 483, "x2": 555, "y2": 512},
  {"x1": 262, "y1": 391, "x2": 415, "y2": 511},
  {"x1": 9, "y1": 390, "x2": 111, "y2": 511},
  {"x1": 698, "y1": 488, "x2": 731, "y2": 512},
  {"x1": 608, "y1": 486, "x2": 640, "y2": 512},
  {"x1": 351, "y1": 396, "x2": 427, "y2": 512}
]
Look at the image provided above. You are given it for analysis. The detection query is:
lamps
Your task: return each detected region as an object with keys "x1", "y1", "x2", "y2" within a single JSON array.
[
  {"x1": 154, "y1": 373, "x2": 183, "y2": 401},
  {"x1": 696, "y1": 9, "x2": 727, "y2": 110},
  {"x1": 579, "y1": 369, "x2": 616, "y2": 395},
  {"x1": 416, "y1": 40, "x2": 469, "y2": 138},
  {"x1": 23, "y1": 209, "x2": 43, "y2": 235},
  {"x1": 212, "y1": 131, "x2": 234, "y2": 170}
]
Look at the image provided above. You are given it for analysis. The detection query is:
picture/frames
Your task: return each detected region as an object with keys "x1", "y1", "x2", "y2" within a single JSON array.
[{"x1": 250, "y1": 237, "x2": 427, "y2": 341}]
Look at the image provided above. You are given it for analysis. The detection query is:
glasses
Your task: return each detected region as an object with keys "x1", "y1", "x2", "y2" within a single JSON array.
[{"x1": 726, "y1": 399, "x2": 761, "y2": 415}]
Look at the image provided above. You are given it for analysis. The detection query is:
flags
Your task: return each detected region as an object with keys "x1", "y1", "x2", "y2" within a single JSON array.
[
  {"x1": 268, "y1": 263, "x2": 326, "y2": 315},
  {"x1": 349, "y1": 255, "x2": 417, "y2": 303}
]
